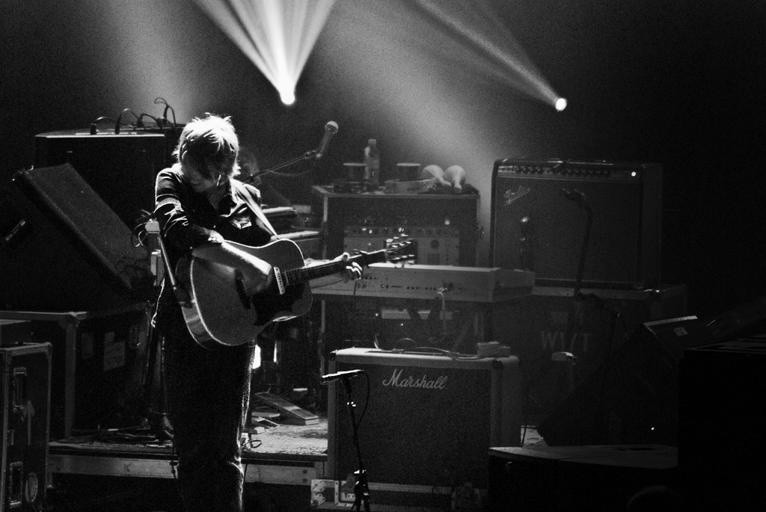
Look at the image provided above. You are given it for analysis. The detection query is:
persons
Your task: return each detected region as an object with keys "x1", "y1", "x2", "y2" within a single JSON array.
[{"x1": 154, "y1": 113, "x2": 362, "y2": 512}]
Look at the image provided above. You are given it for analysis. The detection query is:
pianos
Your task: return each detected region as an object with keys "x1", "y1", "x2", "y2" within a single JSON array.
[{"x1": 304, "y1": 257, "x2": 536, "y2": 304}]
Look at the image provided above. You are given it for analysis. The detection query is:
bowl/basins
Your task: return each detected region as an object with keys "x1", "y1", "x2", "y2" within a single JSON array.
[{"x1": 384, "y1": 178, "x2": 432, "y2": 194}]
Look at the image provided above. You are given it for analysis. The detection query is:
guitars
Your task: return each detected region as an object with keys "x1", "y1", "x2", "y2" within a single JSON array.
[{"x1": 175, "y1": 233, "x2": 416, "y2": 348}]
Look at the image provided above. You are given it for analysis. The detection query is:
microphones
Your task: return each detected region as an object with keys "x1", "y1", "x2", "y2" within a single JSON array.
[
  {"x1": 317, "y1": 119, "x2": 339, "y2": 161},
  {"x1": 319, "y1": 368, "x2": 362, "y2": 384}
]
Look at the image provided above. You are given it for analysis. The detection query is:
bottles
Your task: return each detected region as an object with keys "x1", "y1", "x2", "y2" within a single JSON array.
[{"x1": 361, "y1": 138, "x2": 381, "y2": 189}]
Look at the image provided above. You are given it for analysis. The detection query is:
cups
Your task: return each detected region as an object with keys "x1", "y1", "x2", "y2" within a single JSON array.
[
  {"x1": 341, "y1": 161, "x2": 366, "y2": 193},
  {"x1": 396, "y1": 163, "x2": 420, "y2": 182}
]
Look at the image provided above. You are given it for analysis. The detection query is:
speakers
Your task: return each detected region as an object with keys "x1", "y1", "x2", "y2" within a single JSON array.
[
  {"x1": 325, "y1": 347, "x2": 522, "y2": 493},
  {"x1": 35, "y1": 125, "x2": 182, "y2": 247},
  {"x1": 488, "y1": 159, "x2": 646, "y2": 290},
  {"x1": 0, "y1": 162, "x2": 158, "y2": 312}
]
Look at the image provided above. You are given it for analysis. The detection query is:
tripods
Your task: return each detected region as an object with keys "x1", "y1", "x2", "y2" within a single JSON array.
[{"x1": 70, "y1": 320, "x2": 172, "y2": 444}]
